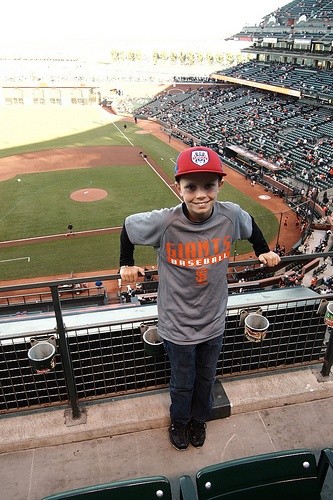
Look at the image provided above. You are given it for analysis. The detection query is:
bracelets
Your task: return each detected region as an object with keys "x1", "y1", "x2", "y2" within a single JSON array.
[{"x1": 121, "y1": 265, "x2": 128, "y2": 269}]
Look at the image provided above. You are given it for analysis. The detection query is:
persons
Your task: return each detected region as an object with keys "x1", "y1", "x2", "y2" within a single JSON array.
[
  {"x1": 120, "y1": 147, "x2": 281, "y2": 452},
  {"x1": 64, "y1": 0, "x2": 333, "y2": 305}
]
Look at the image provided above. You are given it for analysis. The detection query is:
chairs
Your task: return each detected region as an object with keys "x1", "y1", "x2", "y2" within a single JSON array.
[
  {"x1": 134, "y1": 0, "x2": 333, "y2": 304},
  {"x1": 41, "y1": 448, "x2": 333, "y2": 500}
]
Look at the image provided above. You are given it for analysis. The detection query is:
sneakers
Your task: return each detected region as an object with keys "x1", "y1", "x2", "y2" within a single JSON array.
[
  {"x1": 167, "y1": 419, "x2": 191, "y2": 451},
  {"x1": 189, "y1": 419, "x2": 208, "y2": 449}
]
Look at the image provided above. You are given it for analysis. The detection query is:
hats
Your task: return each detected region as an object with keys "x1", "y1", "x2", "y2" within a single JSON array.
[{"x1": 173, "y1": 145, "x2": 227, "y2": 180}]
